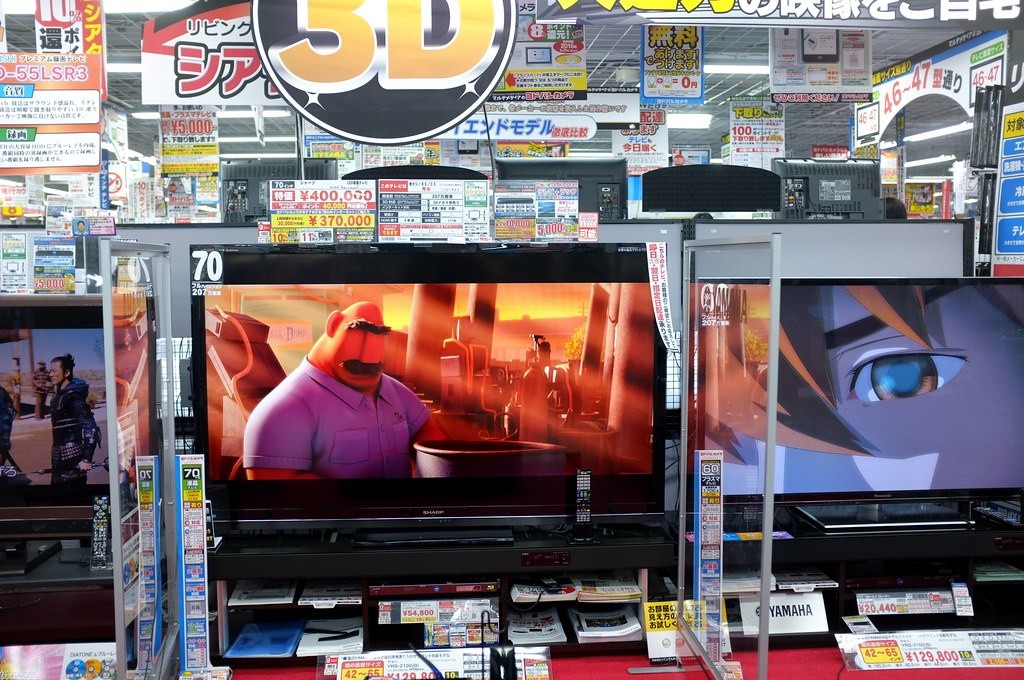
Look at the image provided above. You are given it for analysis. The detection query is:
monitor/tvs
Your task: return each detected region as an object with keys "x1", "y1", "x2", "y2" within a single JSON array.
[
  {"x1": 217, "y1": 158, "x2": 338, "y2": 225},
  {"x1": 771, "y1": 157, "x2": 887, "y2": 222},
  {"x1": 685, "y1": 278, "x2": 1024, "y2": 532},
  {"x1": 496, "y1": 157, "x2": 629, "y2": 223},
  {"x1": 0, "y1": 292, "x2": 160, "y2": 577},
  {"x1": 190, "y1": 244, "x2": 683, "y2": 550}
]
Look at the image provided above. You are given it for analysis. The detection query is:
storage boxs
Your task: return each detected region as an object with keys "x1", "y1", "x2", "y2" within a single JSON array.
[
  {"x1": 0, "y1": 54, "x2": 222, "y2": 227},
  {"x1": 0, "y1": 232, "x2": 76, "y2": 294}
]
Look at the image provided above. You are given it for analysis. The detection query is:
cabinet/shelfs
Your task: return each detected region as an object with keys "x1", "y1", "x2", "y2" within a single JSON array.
[
  {"x1": 0, "y1": 241, "x2": 177, "y2": 644},
  {"x1": 210, "y1": 515, "x2": 1024, "y2": 666}
]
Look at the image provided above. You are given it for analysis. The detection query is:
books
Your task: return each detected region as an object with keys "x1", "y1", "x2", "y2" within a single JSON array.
[
  {"x1": 722, "y1": 570, "x2": 777, "y2": 592},
  {"x1": 508, "y1": 574, "x2": 643, "y2": 644}
]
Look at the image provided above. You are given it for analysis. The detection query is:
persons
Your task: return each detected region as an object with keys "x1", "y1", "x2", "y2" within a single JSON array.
[
  {"x1": 48, "y1": 352, "x2": 102, "y2": 485},
  {"x1": 886, "y1": 197, "x2": 908, "y2": 219},
  {"x1": 0, "y1": 385, "x2": 16, "y2": 468},
  {"x1": 31, "y1": 361, "x2": 53, "y2": 419}
]
